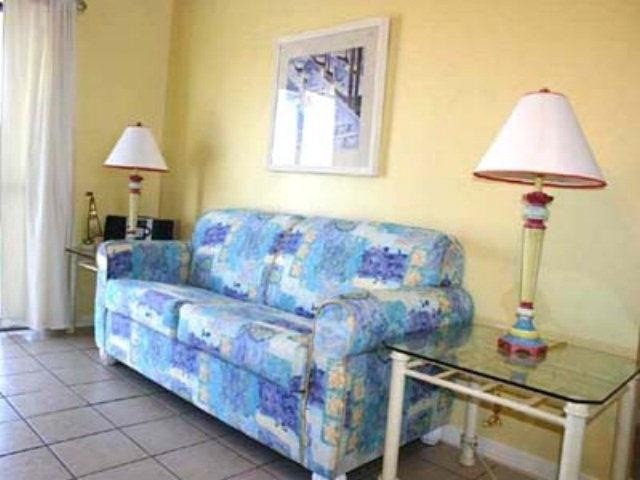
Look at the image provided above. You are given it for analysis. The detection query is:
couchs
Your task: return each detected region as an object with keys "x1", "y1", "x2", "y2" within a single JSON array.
[{"x1": 91, "y1": 206, "x2": 476, "y2": 480}]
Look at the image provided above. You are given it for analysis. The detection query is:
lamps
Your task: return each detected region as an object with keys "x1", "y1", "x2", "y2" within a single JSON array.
[
  {"x1": 471, "y1": 88, "x2": 609, "y2": 359},
  {"x1": 101, "y1": 122, "x2": 172, "y2": 240}
]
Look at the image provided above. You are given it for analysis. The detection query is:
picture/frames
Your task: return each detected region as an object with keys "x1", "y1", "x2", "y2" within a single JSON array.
[{"x1": 267, "y1": 15, "x2": 390, "y2": 178}]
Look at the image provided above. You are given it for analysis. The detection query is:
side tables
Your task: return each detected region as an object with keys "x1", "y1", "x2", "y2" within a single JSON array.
[
  {"x1": 379, "y1": 320, "x2": 640, "y2": 480},
  {"x1": 65, "y1": 246, "x2": 96, "y2": 334}
]
